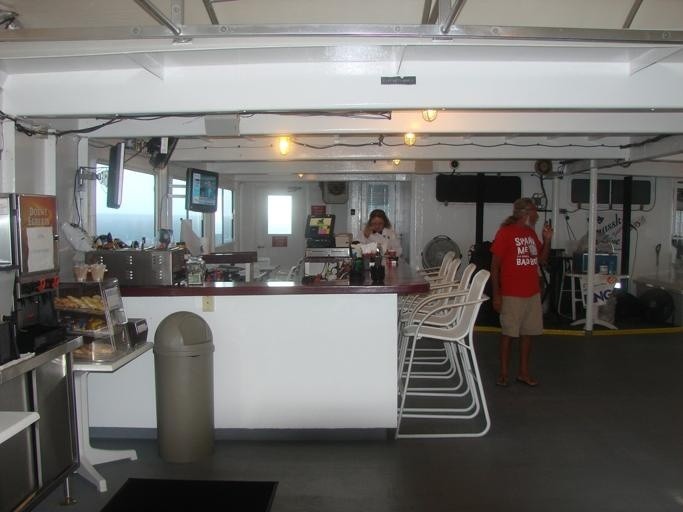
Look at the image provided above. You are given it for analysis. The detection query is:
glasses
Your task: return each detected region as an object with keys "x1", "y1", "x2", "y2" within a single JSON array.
[
  {"x1": 392, "y1": 108, "x2": 438, "y2": 165},
  {"x1": 277, "y1": 136, "x2": 290, "y2": 156},
  {"x1": 297, "y1": 173, "x2": 304, "y2": 179}
]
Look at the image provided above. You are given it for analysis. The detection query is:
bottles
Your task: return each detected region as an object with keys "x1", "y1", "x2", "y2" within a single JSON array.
[{"x1": 514, "y1": 198, "x2": 535, "y2": 219}]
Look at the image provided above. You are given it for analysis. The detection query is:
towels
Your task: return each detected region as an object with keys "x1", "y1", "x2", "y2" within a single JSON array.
[{"x1": 152, "y1": 310, "x2": 214, "y2": 465}]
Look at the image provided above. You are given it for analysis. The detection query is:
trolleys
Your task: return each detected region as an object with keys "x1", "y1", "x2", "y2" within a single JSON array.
[{"x1": 421, "y1": 235, "x2": 462, "y2": 277}]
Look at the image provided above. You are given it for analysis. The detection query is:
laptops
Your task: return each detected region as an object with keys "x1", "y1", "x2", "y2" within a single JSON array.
[
  {"x1": 54, "y1": 293, "x2": 106, "y2": 311},
  {"x1": 86, "y1": 318, "x2": 105, "y2": 329}
]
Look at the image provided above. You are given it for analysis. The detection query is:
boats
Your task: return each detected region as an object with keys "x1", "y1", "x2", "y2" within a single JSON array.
[{"x1": 435, "y1": 173, "x2": 522, "y2": 205}]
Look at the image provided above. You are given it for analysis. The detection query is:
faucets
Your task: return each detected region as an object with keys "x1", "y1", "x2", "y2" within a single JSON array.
[
  {"x1": 516, "y1": 374, "x2": 544, "y2": 386},
  {"x1": 495, "y1": 375, "x2": 508, "y2": 385}
]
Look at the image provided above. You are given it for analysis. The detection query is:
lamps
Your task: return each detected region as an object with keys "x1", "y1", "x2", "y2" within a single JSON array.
[
  {"x1": 557, "y1": 240, "x2": 581, "y2": 321},
  {"x1": 267, "y1": 257, "x2": 305, "y2": 281},
  {"x1": 394, "y1": 250, "x2": 492, "y2": 441}
]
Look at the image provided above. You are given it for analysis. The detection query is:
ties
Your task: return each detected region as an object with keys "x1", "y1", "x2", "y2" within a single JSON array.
[
  {"x1": 611, "y1": 179, "x2": 651, "y2": 204},
  {"x1": 148, "y1": 135, "x2": 182, "y2": 172},
  {"x1": 571, "y1": 178, "x2": 610, "y2": 204},
  {"x1": 106, "y1": 141, "x2": 127, "y2": 210},
  {"x1": 185, "y1": 166, "x2": 220, "y2": 213}
]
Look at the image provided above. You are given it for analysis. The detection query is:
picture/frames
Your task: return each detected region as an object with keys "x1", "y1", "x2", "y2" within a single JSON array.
[
  {"x1": 570, "y1": 274, "x2": 631, "y2": 330},
  {"x1": 51, "y1": 341, "x2": 155, "y2": 493}
]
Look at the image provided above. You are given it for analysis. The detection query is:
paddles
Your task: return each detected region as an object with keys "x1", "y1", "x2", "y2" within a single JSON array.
[{"x1": 98, "y1": 478, "x2": 279, "y2": 512}]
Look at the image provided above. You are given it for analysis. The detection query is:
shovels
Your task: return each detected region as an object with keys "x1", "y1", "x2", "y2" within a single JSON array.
[{"x1": 73, "y1": 342, "x2": 114, "y2": 359}]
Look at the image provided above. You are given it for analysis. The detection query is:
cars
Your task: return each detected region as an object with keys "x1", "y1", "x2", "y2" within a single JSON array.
[
  {"x1": 599, "y1": 266, "x2": 609, "y2": 274},
  {"x1": 71, "y1": 263, "x2": 90, "y2": 283},
  {"x1": 90, "y1": 263, "x2": 108, "y2": 283},
  {"x1": 369, "y1": 265, "x2": 385, "y2": 281}
]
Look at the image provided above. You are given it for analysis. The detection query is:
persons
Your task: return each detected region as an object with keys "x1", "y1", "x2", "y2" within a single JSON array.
[
  {"x1": 489, "y1": 196, "x2": 553, "y2": 387},
  {"x1": 352, "y1": 209, "x2": 402, "y2": 266}
]
